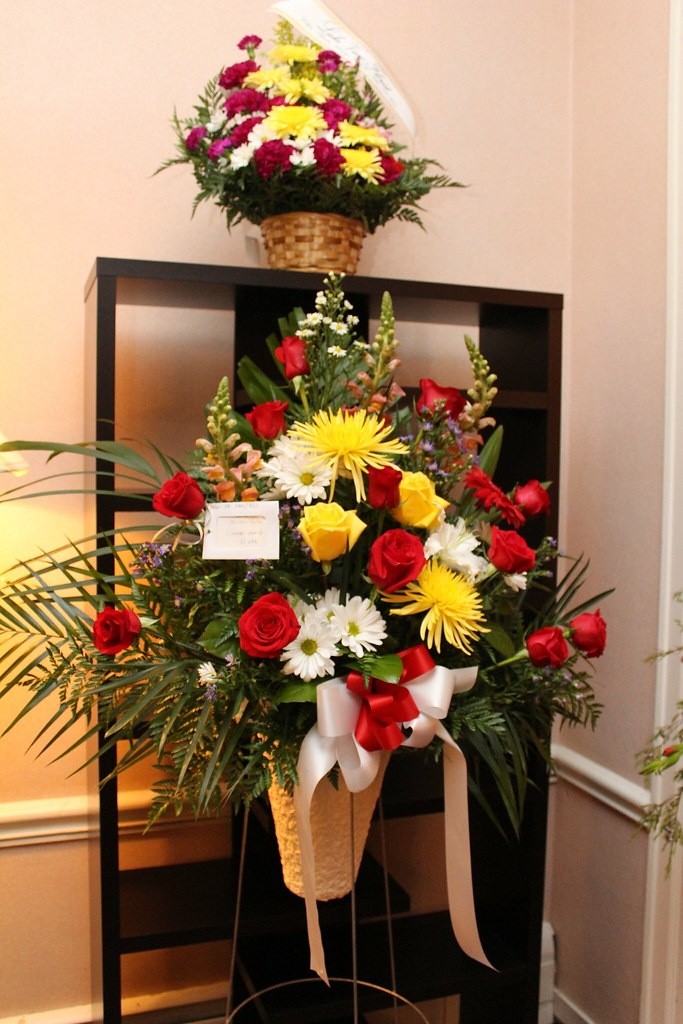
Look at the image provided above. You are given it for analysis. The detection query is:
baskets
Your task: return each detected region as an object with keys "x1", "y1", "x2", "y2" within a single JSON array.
[{"x1": 261, "y1": 213, "x2": 364, "y2": 274}]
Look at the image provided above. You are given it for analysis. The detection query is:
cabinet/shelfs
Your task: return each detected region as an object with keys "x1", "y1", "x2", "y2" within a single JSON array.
[{"x1": 77, "y1": 256, "x2": 567, "y2": 1022}]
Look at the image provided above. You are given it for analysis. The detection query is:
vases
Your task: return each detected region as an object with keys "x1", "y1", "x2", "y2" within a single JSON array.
[
  {"x1": 220, "y1": 694, "x2": 404, "y2": 909},
  {"x1": 255, "y1": 208, "x2": 372, "y2": 274}
]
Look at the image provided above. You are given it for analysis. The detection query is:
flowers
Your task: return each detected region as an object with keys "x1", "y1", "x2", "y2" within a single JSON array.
[
  {"x1": 0, "y1": 275, "x2": 608, "y2": 814},
  {"x1": 147, "y1": 0, "x2": 474, "y2": 236}
]
